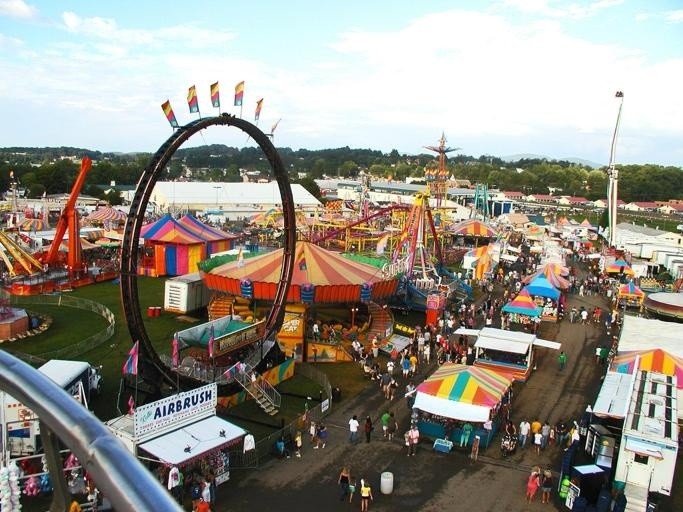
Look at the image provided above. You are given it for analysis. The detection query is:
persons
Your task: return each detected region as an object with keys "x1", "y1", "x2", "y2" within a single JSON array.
[
  {"x1": 352, "y1": 325, "x2": 484, "y2": 409},
  {"x1": 559, "y1": 238, "x2": 632, "y2": 325},
  {"x1": 278, "y1": 421, "x2": 328, "y2": 459},
  {"x1": 69, "y1": 499, "x2": 81, "y2": 512},
  {"x1": 560, "y1": 345, "x2": 608, "y2": 367},
  {"x1": 439, "y1": 266, "x2": 553, "y2": 333},
  {"x1": 348, "y1": 413, "x2": 420, "y2": 456},
  {"x1": 423, "y1": 412, "x2": 579, "y2": 458},
  {"x1": 190, "y1": 480, "x2": 216, "y2": 512},
  {"x1": 456, "y1": 237, "x2": 539, "y2": 275},
  {"x1": 310, "y1": 318, "x2": 345, "y2": 343},
  {"x1": 525, "y1": 465, "x2": 555, "y2": 502},
  {"x1": 338, "y1": 466, "x2": 374, "y2": 512},
  {"x1": 190, "y1": 488, "x2": 208, "y2": 512}
]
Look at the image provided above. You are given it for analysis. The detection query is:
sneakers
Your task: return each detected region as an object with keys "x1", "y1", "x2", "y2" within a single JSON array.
[
  {"x1": 295, "y1": 451, "x2": 301, "y2": 457},
  {"x1": 313, "y1": 443, "x2": 325, "y2": 449}
]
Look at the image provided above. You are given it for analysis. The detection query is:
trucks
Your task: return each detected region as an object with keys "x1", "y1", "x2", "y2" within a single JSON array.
[{"x1": 0, "y1": 360, "x2": 104, "y2": 457}]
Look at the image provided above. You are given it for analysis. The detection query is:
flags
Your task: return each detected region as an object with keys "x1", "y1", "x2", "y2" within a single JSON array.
[
  {"x1": 208, "y1": 325, "x2": 213, "y2": 358},
  {"x1": 161, "y1": 99, "x2": 177, "y2": 127},
  {"x1": 121, "y1": 340, "x2": 139, "y2": 376},
  {"x1": 234, "y1": 81, "x2": 245, "y2": 107},
  {"x1": 271, "y1": 118, "x2": 281, "y2": 133},
  {"x1": 210, "y1": 81, "x2": 220, "y2": 107},
  {"x1": 185, "y1": 85, "x2": 199, "y2": 114},
  {"x1": 254, "y1": 98, "x2": 263, "y2": 120},
  {"x1": 171, "y1": 330, "x2": 180, "y2": 367}
]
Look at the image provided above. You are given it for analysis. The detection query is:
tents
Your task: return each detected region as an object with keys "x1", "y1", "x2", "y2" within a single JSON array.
[
  {"x1": 500, "y1": 261, "x2": 570, "y2": 329},
  {"x1": 413, "y1": 363, "x2": 514, "y2": 425},
  {"x1": 606, "y1": 257, "x2": 644, "y2": 312}
]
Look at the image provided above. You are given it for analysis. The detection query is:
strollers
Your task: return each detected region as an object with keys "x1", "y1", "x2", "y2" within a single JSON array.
[{"x1": 500, "y1": 434, "x2": 518, "y2": 457}]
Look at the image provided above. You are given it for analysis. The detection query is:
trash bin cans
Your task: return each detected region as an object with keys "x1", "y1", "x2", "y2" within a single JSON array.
[
  {"x1": 332, "y1": 388, "x2": 342, "y2": 402},
  {"x1": 380, "y1": 470, "x2": 394, "y2": 495},
  {"x1": 148, "y1": 307, "x2": 155, "y2": 317},
  {"x1": 572, "y1": 497, "x2": 588, "y2": 512},
  {"x1": 156, "y1": 306, "x2": 162, "y2": 317}
]
